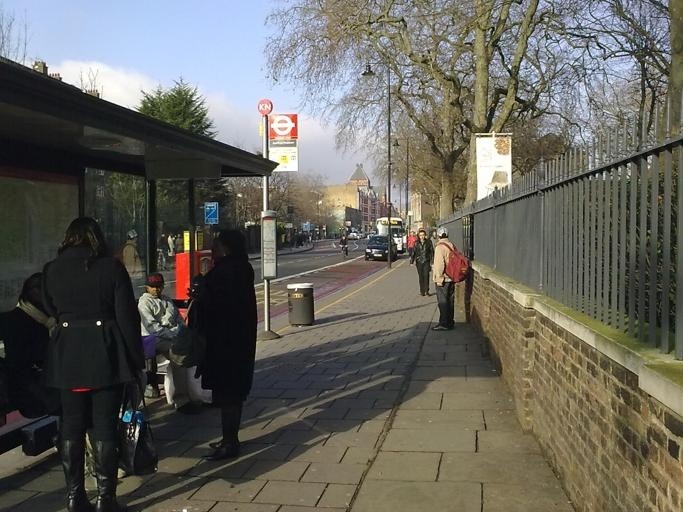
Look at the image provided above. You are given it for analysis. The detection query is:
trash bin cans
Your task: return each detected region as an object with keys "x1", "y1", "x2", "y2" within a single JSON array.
[{"x1": 286, "y1": 283, "x2": 314, "y2": 327}]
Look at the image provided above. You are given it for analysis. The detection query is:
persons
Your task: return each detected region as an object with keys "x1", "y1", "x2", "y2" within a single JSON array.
[
  {"x1": 136, "y1": 272, "x2": 214, "y2": 416},
  {"x1": 338, "y1": 235, "x2": 350, "y2": 256},
  {"x1": 430, "y1": 226, "x2": 458, "y2": 332},
  {"x1": 184, "y1": 226, "x2": 261, "y2": 465},
  {"x1": 427, "y1": 229, "x2": 437, "y2": 246},
  {"x1": 41, "y1": 214, "x2": 145, "y2": 512},
  {"x1": 120, "y1": 227, "x2": 143, "y2": 302},
  {"x1": 157, "y1": 231, "x2": 184, "y2": 271},
  {"x1": 406, "y1": 229, "x2": 417, "y2": 260},
  {"x1": 0, "y1": 269, "x2": 128, "y2": 485},
  {"x1": 408, "y1": 229, "x2": 435, "y2": 297}
]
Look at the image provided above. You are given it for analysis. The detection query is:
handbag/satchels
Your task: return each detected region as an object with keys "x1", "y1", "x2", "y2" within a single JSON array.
[
  {"x1": 117, "y1": 377, "x2": 158, "y2": 475},
  {"x1": 169, "y1": 300, "x2": 205, "y2": 369}
]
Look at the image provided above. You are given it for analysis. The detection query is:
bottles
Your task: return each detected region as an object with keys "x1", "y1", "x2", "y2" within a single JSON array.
[{"x1": 135, "y1": 411, "x2": 146, "y2": 436}]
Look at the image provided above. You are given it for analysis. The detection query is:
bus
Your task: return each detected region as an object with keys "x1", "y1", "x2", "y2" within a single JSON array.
[{"x1": 375, "y1": 216, "x2": 403, "y2": 255}]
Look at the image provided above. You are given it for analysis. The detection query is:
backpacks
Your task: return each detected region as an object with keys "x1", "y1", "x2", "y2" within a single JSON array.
[{"x1": 435, "y1": 240, "x2": 471, "y2": 282}]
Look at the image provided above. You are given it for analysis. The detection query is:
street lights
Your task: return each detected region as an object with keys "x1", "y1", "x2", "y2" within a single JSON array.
[
  {"x1": 359, "y1": 49, "x2": 391, "y2": 268},
  {"x1": 392, "y1": 137, "x2": 408, "y2": 222}
]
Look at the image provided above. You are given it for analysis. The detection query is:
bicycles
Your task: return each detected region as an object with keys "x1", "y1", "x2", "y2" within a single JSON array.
[
  {"x1": 157, "y1": 247, "x2": 167, "y2": 271},
  {"x1": 341, "y1": 245, "x2": 348, "y2": 259}
]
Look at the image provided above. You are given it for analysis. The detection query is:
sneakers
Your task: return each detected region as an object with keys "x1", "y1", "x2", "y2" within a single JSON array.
[{"x1": 431, "y1": 325, "x2": 449, "y2": 330}]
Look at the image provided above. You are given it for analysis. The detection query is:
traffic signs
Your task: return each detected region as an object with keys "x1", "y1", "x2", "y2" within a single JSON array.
[{"x1": 205, "y1": 202, "x2": 218, "y2": 224}]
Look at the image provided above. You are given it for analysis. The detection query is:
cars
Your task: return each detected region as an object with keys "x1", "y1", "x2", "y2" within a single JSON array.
[
  {"x1": 363, "y1": 236, "x2": 397, "y2": 262},
  {"x1": 348, "y1": 228, "x2": 407, "y2": 249}
]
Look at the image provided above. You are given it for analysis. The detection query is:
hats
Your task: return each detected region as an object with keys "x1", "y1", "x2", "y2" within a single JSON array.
[
  {"x1": 144, "y1": 273, "x2": 164, "y2": 287},
  {"x1": 437, "y1": 227, "x2": 449, "y2": 237}
]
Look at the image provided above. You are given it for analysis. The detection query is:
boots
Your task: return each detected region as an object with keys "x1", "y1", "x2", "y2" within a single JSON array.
[
  {"x1": 56, "y1": 436, "x2": 94, "y2": 511},
  {"x1": 91, "y1": 437, "x2": 125, "y2": 512}
]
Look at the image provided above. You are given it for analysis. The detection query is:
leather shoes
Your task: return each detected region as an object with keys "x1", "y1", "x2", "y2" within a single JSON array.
[{"x1": 201, "y1": 440, "x2": 237, "y2": 460}]
